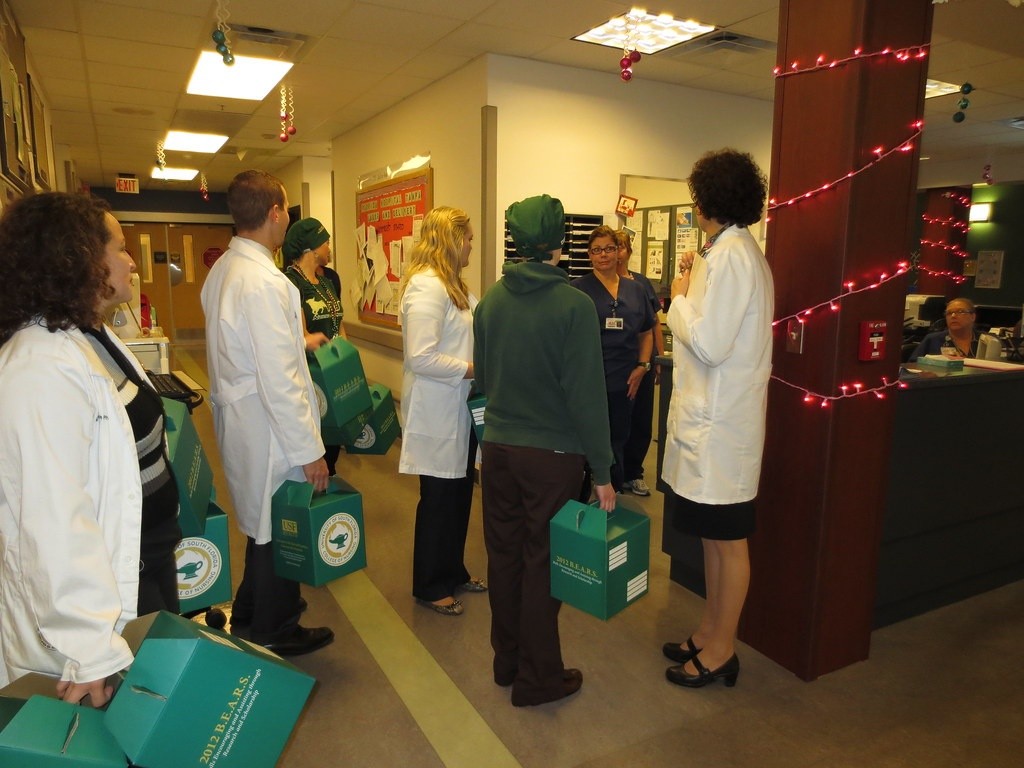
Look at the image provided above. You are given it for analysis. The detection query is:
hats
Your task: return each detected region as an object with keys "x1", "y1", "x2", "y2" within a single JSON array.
[
  {"x1": 282, "y1": 218, "x2": 330, "y2": 258},
  {"x1": 507, "y1": 194, "x2": 565, "y2": 262}
]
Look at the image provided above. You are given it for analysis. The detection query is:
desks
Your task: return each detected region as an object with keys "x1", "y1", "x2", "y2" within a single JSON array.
[{"x1": 655, "y1": 358, "x2": 1024, "y2": 630}]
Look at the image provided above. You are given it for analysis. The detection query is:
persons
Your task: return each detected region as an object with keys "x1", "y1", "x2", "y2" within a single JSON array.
[
  {"x1": 280, "y1": 243, "x2": 341, "y2": 297},
  {"x1": 662, "y1": 148, "x2": 776, "y2": 688},
  {"x1": 397, "y1": 206, "x2": 489, "y2": 614},
  {"x1": 0, "y1": 191, "x2": 182, "y2": 711},
  {"x1": 570, "y1": 224, "x2": 656, "y2": 498},
  {"x1": 286, "y1": 218, "x2": 343, "y2": 475},
  {"x1": 612, "y1": 229, "x2": 664, "y2": 356},
  {"x1": 471, "y1": 194, "x2": 616, "y2": 706},
  {"x1": 200, "y1": 170, "x2": 335, "y2": 660}
]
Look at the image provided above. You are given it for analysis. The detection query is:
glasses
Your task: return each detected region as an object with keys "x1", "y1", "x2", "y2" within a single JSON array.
[
  {"x1": 588, "y1": 246, "x2": 618, "y2": 255},
  {"x1": 946, "y1": 310, "x2": 969, "y2": 317}
]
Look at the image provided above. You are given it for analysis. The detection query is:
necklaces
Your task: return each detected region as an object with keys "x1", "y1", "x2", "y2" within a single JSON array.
[{"x1": 294, "y1": 261, "x2": 339, "y2": 337}]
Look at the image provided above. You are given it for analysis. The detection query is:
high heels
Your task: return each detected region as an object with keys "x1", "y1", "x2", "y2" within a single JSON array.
[{"x1": 663, "y1": 635, "x2": 740, "y2": 687}]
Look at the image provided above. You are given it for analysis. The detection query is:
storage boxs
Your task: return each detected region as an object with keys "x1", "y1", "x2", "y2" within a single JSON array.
[
  {"x1": 158, "y1": 396, "x2": 215, "y2": 537},
  {"x1": 466, "y1": 379, "x2": 487, "y2": 450},
  {"x1": 305, "y1": 335, "x2": 400, "y2": 455},
  {"x1": 0, "y1": 610, "x2": 317, "y2": 768},
  {"x1": 271, "y1": 474, "x2": 368, "y2": 589},
  {"x1": 549, "y1": 491, "x2": 651, "y2": 621},
  {"x1": 173, "y1": 499, "x2": 232, "y2": 614}
]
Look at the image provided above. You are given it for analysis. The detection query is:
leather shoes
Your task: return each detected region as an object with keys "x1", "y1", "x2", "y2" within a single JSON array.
[
  {"x1": 230, "y1": 597, "x2": 307, "y2": 625},
  {"x1": 511, "y1": 668, "x2": 583, "y2": 707},
  {"x1": 458, "y1": 576, "x2": 488, "y2": 592},
  {"x1": 250, "y1": 624, "x2": 334, "y2": 655},
  {"x1": 493, "y1": 672, "x2": 514, "y2": 686},
  {"x1": 415, "y1": 598, "x2": 463, "y2": 615}
]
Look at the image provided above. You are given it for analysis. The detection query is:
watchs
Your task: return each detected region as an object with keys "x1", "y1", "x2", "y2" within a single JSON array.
[{"x1": 637, "y1": 361, "x2": 650, "y2": 371}]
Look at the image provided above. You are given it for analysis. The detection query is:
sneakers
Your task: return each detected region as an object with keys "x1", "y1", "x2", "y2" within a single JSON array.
[{"x1": 622, "y1": 479, "x2": 651, "y2": 496}]
